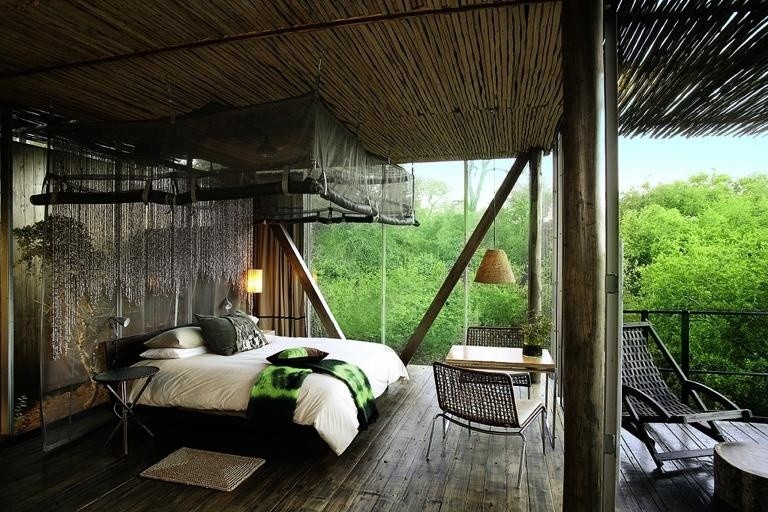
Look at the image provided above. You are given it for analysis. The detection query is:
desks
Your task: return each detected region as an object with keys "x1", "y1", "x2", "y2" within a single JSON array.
[
  {"x1": 445, "y1": 343, "x2": 558, "y2": 449},
  {"x1": 93, "y1": 366, "x2": 159, "y2": 456},
  {"x1": 715, "y1": 441, "x2": 768, "y2": 511},
  {"x1": 263, "y1": 330, "x2": 275, "y2": 335}
]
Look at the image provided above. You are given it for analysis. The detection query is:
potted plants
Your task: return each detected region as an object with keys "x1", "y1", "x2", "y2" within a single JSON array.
[{"x1": 519, "y1": 310, "x2": 554, "y2": 356}]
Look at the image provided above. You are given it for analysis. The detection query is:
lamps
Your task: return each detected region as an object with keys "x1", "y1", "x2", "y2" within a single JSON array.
[
  {"x1": 108, "y1": 314, "x2": 131, "y2": 337},
  {"x1": 247, "y1": 269, "x2": 263, "y2": 293},
  {"x1": 223, "y1": 285, "x2": 233, "y2": 311},
  {"x1": 474, "y1": 126, "x2": 516, "y2": 285}
]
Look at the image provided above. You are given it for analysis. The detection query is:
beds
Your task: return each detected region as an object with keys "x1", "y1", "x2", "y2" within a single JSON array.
[{"x1": 103, "y1": 324, "x2": 409, "y2": 456}]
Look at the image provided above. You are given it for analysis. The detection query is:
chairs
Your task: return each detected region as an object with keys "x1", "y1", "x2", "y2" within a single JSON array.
[
  {"x1": 426, "y1": 362, "x2": 547, "y2": 488},
  {"x1": 467, "y1": 326, "x2": 531, "y2": 399},
  {"x1": 622, "y1": 321, "x2": 753, "y2": 478}
]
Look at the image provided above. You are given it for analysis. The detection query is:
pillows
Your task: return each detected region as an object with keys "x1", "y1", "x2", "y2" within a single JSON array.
[
  {"x1": 265, "y1": 347, "x2": 329, "y2": 366},
  {"x1": 140, "y1": 310, "x2": 273, "y2": 358}
]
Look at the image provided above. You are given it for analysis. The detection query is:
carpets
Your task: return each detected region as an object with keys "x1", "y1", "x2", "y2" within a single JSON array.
[{"x1": 141, "y1": 445, "x2": 265, "y2": 492}]
